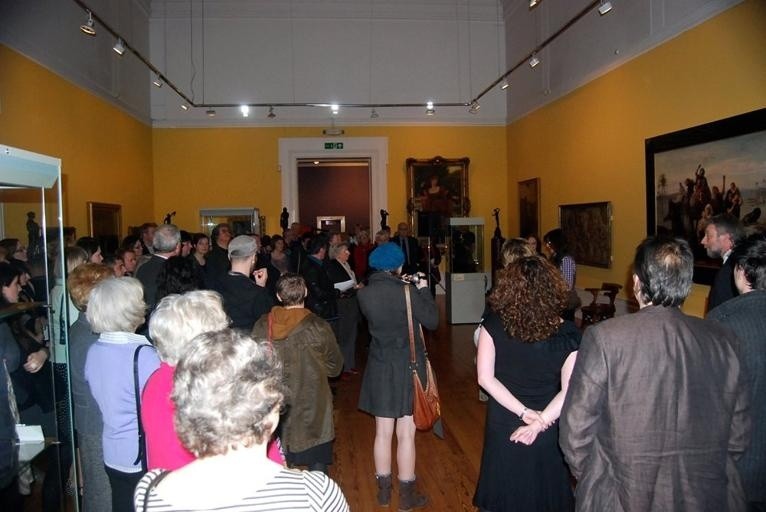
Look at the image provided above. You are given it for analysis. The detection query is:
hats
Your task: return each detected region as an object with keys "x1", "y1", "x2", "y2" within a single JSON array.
[
  {"x1": 548, "y1": 229, "x2": 570, "y2": 251},
  {"x1": 227, "y1": 235, "x2": 261, "y2": 261},
  {"x1": 368, "y1": 242, "x2": 406, "y2": 273}
]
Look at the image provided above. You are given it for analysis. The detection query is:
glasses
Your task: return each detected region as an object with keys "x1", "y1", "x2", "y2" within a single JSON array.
[{"x1": 14, "y1": 246, "x2": 25, "y2": 253}]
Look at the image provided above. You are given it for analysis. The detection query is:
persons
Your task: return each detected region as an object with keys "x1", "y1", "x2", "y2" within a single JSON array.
[
  {"x1": 163, "y1": 211, "x2": 177, "y2": 225},
  {"x1": 27, "y1": 211, "x2": 40, "y2": 260},
  {"x1": 1, "y1": 216, "x2": 442, "y2": 512},
  {"x1": 380, "y1": 207, "x2": 389, "y2": 230},
  {"x1": 664, "y1": 164, "x2": 762, "y2": 236},
  {"x1": 280, "y1": 207, "x2": 290, "y2": 232},
  {"x1": 450, "y1": 220, "x2": 766, "y2": 511},
  {"x1": 491, "y1": 208, "x2": 502, "y2": 226},
  {"x1": 419, "y1": 170, "x2": 452, "y2": 216}
]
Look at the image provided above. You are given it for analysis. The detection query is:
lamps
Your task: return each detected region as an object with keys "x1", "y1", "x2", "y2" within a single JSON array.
[
  {"x1": 468, "y1": 1, "x2": 615, "y2": 117},
  {"x1": 79, "y1": 11, "x2": 197, "y2": 118},
  {"x1": 199, "y1": 100, "x2": 437, "y2": 119}
]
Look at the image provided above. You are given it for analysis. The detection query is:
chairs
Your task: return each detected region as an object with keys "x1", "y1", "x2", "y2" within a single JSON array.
[{"x1": 580, "y1": 282, "x2": 623, "y2": 329}]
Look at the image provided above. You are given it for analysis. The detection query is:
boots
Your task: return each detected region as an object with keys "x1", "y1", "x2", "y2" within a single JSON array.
[
  {"x1": 396, "y1": 473, "x2": 429, "y2": 511},
  {"x1": 374, "y1": 472, "x2": 393, "y2": 507}
]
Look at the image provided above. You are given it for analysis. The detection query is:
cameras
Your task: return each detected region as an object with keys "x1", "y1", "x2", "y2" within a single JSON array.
[{"x1": 410, "y1": 274, "x2": 421, "y2": 284}]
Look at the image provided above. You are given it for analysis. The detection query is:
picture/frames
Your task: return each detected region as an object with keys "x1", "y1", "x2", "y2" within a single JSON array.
[
  {"x1": 405, "y1": 153, "x2": 472, "y2": 255},
  {"x1": 558, "y1": 201, "x2": 614, "y2": 270},
  {"x1": 517, "y1": 176, "x2": 542, "y2": 243},
  {"x1": 85, "y1": 201, "x2": 124, "y2": 250},
  {"x1": 643, "y1": 107, "x2": 766, "y2": 286}
]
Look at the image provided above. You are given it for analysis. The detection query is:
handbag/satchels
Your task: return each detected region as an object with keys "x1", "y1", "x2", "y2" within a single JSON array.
[
  {"x1": 60, "y1": 318, "x2": 65, "y2": 345},
  {"x1": 411, "y1": 356, "x2": 442, "y2": 430}
]
